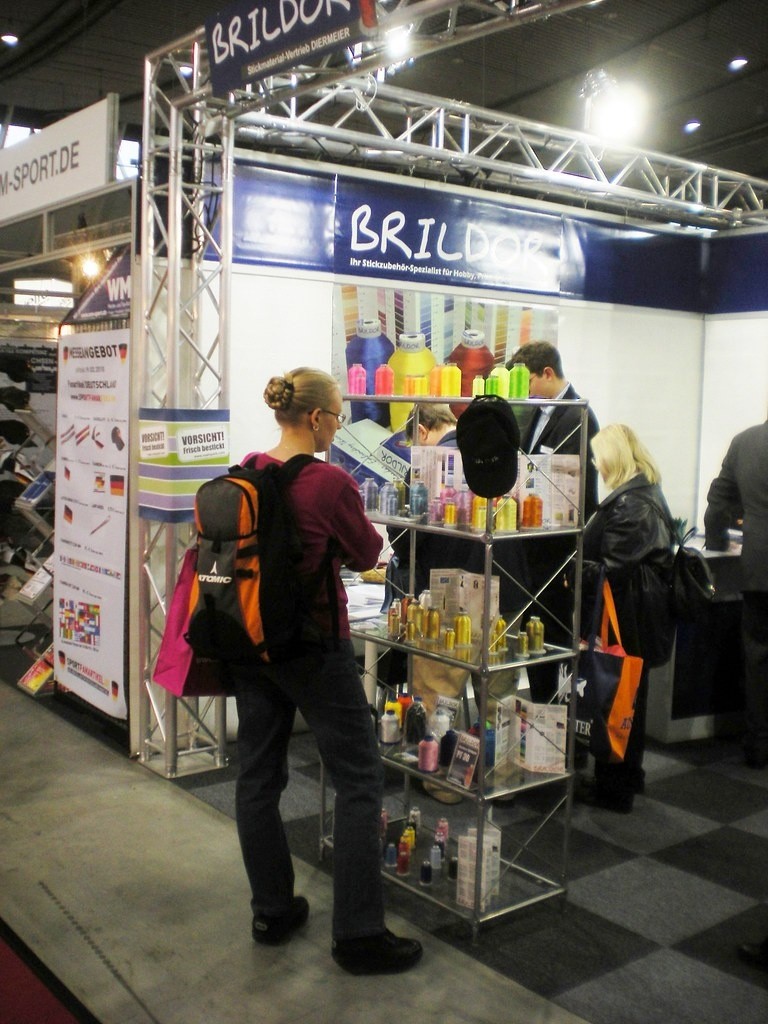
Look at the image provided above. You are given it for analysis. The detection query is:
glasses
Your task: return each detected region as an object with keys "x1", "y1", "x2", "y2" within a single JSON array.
[{"x1": 308, "y1": 409, "x2": 346, "y2": 423}]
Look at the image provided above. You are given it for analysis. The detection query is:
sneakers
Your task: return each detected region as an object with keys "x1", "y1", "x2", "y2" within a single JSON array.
[
  {"x1": 328, "y1": 928, "x2": 424, "y2": 973},
  {"x1": 251, "y1": 896, "x2": 310, "y2": 943}
]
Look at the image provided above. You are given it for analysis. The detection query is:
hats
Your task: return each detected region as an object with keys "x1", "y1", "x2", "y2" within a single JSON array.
[{"x1": 454, "y1": 393, "x2": 524, "y2": 498}]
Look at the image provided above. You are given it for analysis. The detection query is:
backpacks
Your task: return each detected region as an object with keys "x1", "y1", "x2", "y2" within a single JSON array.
[
  {"x1": 619, "y1": 489, "x2": 714, "y2": 626},
  {"x1": 188, "y1": 451, "x2": 330, "y2": 686}
]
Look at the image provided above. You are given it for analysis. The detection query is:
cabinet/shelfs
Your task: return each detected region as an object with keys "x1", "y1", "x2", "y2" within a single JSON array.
[{"x1": 319, "y1": 390, "x2": 590, "y2": 945}]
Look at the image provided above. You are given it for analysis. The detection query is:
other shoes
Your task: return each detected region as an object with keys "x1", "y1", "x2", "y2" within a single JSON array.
[
  {"x1": 627, "y1": 773, "x2": 646, "y2": 793},
  {"x1": 578, "y1": 786, "x2": 633, "y2": 814}
]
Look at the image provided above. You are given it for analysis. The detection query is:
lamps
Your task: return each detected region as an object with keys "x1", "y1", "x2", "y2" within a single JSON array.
[{"x1": 579, "y1": 65, "x2": 650, "y2": 145}]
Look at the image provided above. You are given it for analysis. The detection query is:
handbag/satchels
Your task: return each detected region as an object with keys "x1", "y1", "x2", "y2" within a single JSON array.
[
  {"x1": 150, "y1": 544, "x2": 234, "y2": 700},
  {"x1": 563, "y1": 564, "x2": 642, "y2": 763}
]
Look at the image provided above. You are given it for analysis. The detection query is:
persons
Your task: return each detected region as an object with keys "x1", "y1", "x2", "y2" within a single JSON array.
[
  {"x1": 234, "y1": 366, "x2": 424, "y2": 977},
  {"x1": 704, "y1": 419, "x2": 768, "y2": 768},
  {"x1": 505, "y1": 341, "x2": 600, "y2": 770},
  {"x1": 385, "y1": 398, "x2": 529, "y2": 804},
  {"x1": 563, "y1": 423, "x2": 676, "y2": 815}
]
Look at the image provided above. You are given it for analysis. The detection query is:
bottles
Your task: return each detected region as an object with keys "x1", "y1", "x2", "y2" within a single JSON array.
[
  {"x1": 359, "y1": 478, "x2": 545, "y2": 533},
  {"x1": 448, "y1": 330, "x2": 494, "y2": 420},
  {"x1": 379, "y1": 692, "x2": 494, "y2": 774},
  {"x1": 344, "y1": 317, "x2": 394, "y2": 428},
  {"x1": 347, "y1": 362, "x2": 530, "y2": 400},
  {"x1": 387, "y1": 332, "x2": 435, "y2": 433},
  {"x1": 380, "y1": 806, "x2": 458, "y2": 887},
  {"x1": 387, "y1": 590, "x2": 546, "y2": 658}
]
